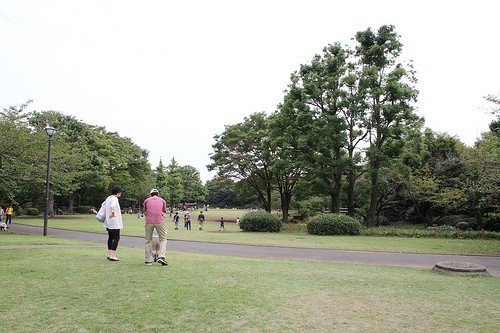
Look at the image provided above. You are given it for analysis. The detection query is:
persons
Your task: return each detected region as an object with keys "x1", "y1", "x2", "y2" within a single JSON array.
[
  {"x1": 219, "y1": 217, "x2": 225, "y2": 231},
  {"x1": 236, "y1": 217, "x2": 241, "y2": 229},
  {"x1": 173, "y1": 212, "x2": 180, "y2": 230},
  {"x1": 144, "y1": 189, "x2": 169, "y2": 265},
  {"x1": 0, "y1": 207, "x2": 6, "y2": 222},
  {"x1": 197, "y1": 211, "x2": 206, "y2": 230},
  {"x1": 184, "y1": 212, "x2": 190, "y2": 228},
  {"x1": 185, "y1": 212, "x2": 192, "y2": 230},
  {"x1": 128, "y1": 203, "x2": 283, "y2": 219},
  {"x1": 6, "y1": 206, "x2": 13, "y2": 223},
  {"x1": 150, "y1": 227, "x2": 161, "y2": 263},
  {"x1": 0, "y1": 223, "x2": 9, "y2": 232},
  {"x1": 101, "y1": 186, "x2": 126, "y2": 261}
]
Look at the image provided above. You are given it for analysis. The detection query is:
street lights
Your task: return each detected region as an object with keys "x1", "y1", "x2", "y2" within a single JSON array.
[{"x1": 43, "y1": 126, "x2": 58, "y2": 236}]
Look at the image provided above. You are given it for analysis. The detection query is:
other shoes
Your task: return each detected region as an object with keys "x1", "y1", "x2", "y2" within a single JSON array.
[
  {"x1": 110, "y1": 257, "x2": 120, "y2": 261},
  {"x1": 144, "y1": 262, "x2": 152, "y2": 265},
  {"x1": 157, "y1": 257, "x2": 168, "y2": 265}
]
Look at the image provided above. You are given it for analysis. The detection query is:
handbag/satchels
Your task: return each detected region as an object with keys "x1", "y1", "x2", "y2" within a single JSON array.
[{"x1": 96, "y1": 202, "x2": 106, "y2": 223}]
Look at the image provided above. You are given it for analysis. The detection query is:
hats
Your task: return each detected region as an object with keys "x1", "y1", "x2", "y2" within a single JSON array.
[
  {"x1": 150, "y1": 188, "x2": 159, "y2": 197},
  {"x1": 112, "y1": 186, "x2": 126, "y2": 194}
]
